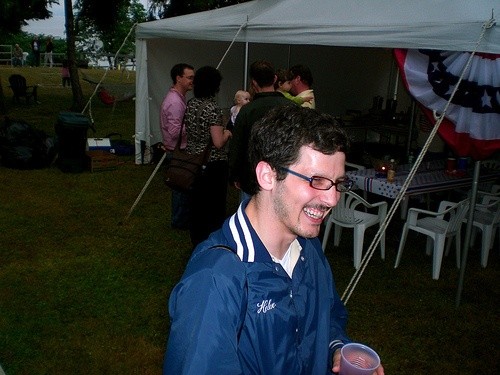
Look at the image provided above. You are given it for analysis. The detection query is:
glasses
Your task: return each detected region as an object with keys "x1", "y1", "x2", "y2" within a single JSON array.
[
  {"x1": 182, "y1": 74, "x2": 193, "y2": 80},
  {"x1": 276, "y1": 164, "x2": 354, "y2": 193}
]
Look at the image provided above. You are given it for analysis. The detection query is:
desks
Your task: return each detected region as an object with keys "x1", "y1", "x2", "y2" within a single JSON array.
[{"x1": 347, "y1": 160, "x2": 500, "y2": 219}]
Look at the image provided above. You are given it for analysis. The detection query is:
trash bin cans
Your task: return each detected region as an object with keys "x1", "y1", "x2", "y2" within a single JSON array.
[{"x1": 55, "y1": 112, "x2": 96, "y2": 174}]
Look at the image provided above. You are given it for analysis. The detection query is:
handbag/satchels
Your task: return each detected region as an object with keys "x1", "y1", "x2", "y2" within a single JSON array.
[
  {"x1": 159, "y1": 147, "x2": 208, "y2": 195},
  {"x1": 0, "y1": 117, "x2": 59, "y2": 169}
]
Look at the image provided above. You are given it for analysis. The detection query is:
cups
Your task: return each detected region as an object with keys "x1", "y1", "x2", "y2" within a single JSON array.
[
  {"x1": 447, "y1": 158, "x2": 456, "y2": 172},
  {"x1": 339, "y1": 342, "x2": 381, "y2": 375},
  {"x1": 459, "y1": 158, "x2": 468, "y2": 170}
]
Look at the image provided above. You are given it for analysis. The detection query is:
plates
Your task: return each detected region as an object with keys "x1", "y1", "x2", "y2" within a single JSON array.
[{"x1": 443, "y1": 170, "x2": 469, "y2": 176}]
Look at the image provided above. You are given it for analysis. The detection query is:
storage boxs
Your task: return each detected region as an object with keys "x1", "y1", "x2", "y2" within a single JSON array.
[
  {"x1": 86, "y1": 138, "x2": 111, "y2": 150},
  {"x1": 86, "y1": 151, "x2": 118, "y2": 173}
]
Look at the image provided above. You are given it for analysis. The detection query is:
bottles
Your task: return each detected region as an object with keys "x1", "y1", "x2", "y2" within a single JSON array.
[
  {"x1": 387, "y1": 159, "x2": 396, "y2": 182},
  {"x1": 408, "y1": 152, "x2": 415, "y2": 173}
]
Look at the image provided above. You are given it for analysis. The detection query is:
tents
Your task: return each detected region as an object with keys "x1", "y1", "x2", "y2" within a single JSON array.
[{"x1": 133, "y1": 0, "x2": 500, "y2": 310}]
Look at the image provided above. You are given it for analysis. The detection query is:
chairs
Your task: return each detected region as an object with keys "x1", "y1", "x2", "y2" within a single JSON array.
[
  {"x1": 8, "y1": 74, "x2": 38, "y2": 104},
  {"x1": 321, "y1": 185, "x2": 387, "y2": 270},
  {"x1": 395, "y1": 185, "x2": 500, "y2": 281}
]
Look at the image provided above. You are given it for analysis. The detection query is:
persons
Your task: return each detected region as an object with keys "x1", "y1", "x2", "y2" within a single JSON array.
[
  {"x1": 159, "y1": 60, "x2": 316, "y2": 241},
  {"x1": 43, "y1": 37, "x2": 53, "y2": 68},
  {"x1": 28, "y1": 35, "x2": 40, "y2": 68},
  {"x1": 62, "y1": 59, "x2": 71, "y2": 87},
  {"x1": 161, "y1": 107, "x2": 385, "y2": 375},
  {"x1": 13, "y1": 43, "x2": 23, "y2": 67}
]
